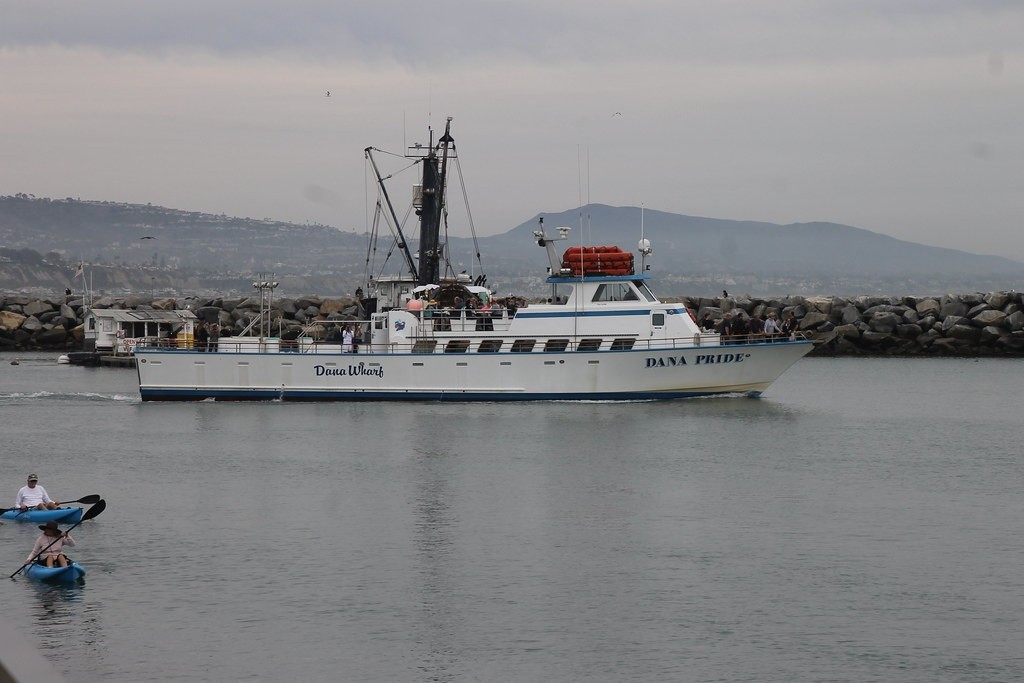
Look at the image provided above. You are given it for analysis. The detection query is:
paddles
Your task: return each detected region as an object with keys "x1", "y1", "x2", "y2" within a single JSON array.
[
  {"x1": 9, "y1": 499, "x2": 107, "y2": 577},
  {"x1": 0, "y1": 494, "x2": 101, "y2": 517}
]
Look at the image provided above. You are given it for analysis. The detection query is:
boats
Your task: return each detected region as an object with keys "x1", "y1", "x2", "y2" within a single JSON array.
[
  {"x1": 23, "y1": 548, "x2": 86, "y2": 586},
  {"x1": 0, "y1": 504, "x2": 84, "y2": 524},
  {"x1": 58, "y1": 305, "x2": 206, "y2": 364},
  {"x1": 128, "y1": 118, "x2": 827, "y2": 403}
]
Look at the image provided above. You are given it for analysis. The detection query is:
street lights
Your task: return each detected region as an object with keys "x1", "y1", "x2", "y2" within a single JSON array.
[
  {"x1": 252, "y1": 271, "x2": 268, "y2": 342},
  {"x1": 263, "y1": 272, "x2": 279, "y2": 339}
]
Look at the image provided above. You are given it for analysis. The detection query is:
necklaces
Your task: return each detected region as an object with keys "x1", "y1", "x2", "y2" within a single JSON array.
[{"x1": 46, "y1": 535, "x2": 54, "y2": 553}]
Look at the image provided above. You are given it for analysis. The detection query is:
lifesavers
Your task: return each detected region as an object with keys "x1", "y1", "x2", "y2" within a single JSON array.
[{"x1": 684, "y1": 306, "x2": 696, "y2": 322}]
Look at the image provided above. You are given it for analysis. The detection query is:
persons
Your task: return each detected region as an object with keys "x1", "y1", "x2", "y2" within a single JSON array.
[
  {"x1": 208, "y1": 324, "x2": 220, "y2": 352},
  {"x1": 197, "y1": 321, "x2": 211, "y2": 351},
  {"x1": 14, "y1": 473, "x2": 72, "y2": 510},
  {"x1": 487, "y1": 296, "x2": 524, "y2": 330},
  {"x1": 622, "y1": 287, "x2": 639, "y2": 300},
  {"x1": 442, "y1": 297, "x2": 488, "y2": 331},
  {"x1": 353, "y1": 323, "x2": 362, "y2": 352},
  {"x1": 341, "y1": 324, "x2": 354, "y2": 353},
  {"x1": 424, "y1": 300, "x2": 441, "y2": 330},
  {"x1": 24, "y1": 522, "x2": 76, "y2": 568},
  {"x1": 698, "y1": 310, "x2": 798, "y2": 344},
  {"x1": 355, "y1": 287, "x2": 363, "y2": 297}
]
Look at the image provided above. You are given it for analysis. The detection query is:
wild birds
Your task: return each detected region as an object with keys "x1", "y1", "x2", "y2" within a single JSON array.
[
  {"x1": 140, "y1": 236, "x2": 160, "y2": 241},
  {"x1": 326, "y1": 91, "x2": 331, "y2": 98},
  {"x1": 65, "y1": 288, "x2": 71, "y2": 295}
]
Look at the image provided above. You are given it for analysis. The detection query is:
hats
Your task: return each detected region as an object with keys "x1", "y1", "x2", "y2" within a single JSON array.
[
  {"x1": 39, "y1": 520, "x2": 62, "y2": 534},
  {"x1": 28, "y1": 474, "x2": 38, "y2": 481}
]
